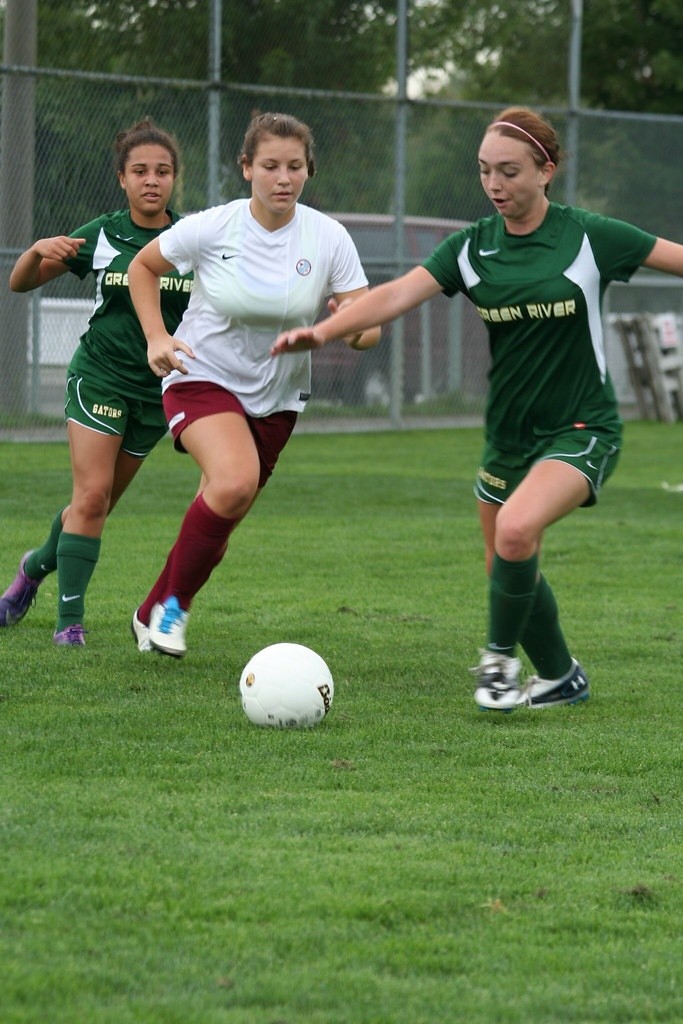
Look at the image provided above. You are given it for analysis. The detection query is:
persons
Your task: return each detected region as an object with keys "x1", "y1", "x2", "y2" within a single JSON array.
[
  {"x1": 128, "y1": 114, "x2": 381, "y2": 657},
  {"x1": 2, "y1": 115, "x2": 196, "y2": 648},
  {"x1": 269, "y1": 106, "x2": 683, "y2": 711}
]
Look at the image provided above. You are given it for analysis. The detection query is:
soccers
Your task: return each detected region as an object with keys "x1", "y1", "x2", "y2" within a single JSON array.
[{"x1": 238, "y1": 644, "x2": 335, "y2": 727}]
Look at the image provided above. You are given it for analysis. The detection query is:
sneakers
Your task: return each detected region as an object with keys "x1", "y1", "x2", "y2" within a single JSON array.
[
  {"x1": 51, "y1": 623, "x2": 87, "y2": 649},
  {"x1": 0, "y1": 549, "x2": 44, "y2": 627},
  {"x1": 469, "y1": 647, "x2": 523, "y2": 714},
  {"x1": 147, "y1": 596, "x2": 190, "y2": 658},
  {"x1": 514, "y1": 656, "x2": 591, "y2": 710},
  {"x1": 131, "y1": 608, "x2": 152, "y2": 655}
]
